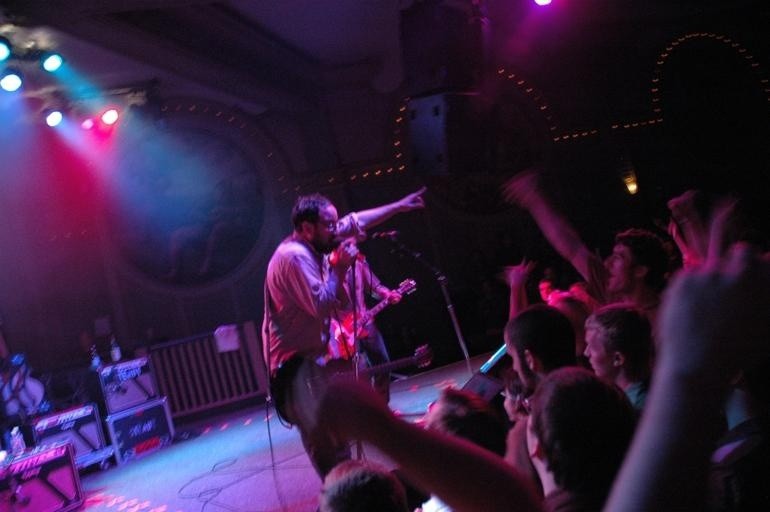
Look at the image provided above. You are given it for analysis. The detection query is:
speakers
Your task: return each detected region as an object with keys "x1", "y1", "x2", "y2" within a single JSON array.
[
  {"x1": 406, "y1": 89, "x2": 475, "y2": 183},
  {"x1": 457, "y1": 370, "x2": 504, "y2": 413}
]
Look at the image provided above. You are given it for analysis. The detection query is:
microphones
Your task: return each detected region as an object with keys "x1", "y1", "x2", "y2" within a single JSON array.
[
  {"x1": 328, "y1": 238, "x2": 341, "y2": 251},
  {"x1": 370, "y1": 229, "x2": 398, "y2": 240}
]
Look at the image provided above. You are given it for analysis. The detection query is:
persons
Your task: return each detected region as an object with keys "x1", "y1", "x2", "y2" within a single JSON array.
[
  {"x1": 314, "y1": 166, "x2": 769, "y2": 512},
  {"x1": 257, "y1": 179, "x2": 432, "y2": 486}
]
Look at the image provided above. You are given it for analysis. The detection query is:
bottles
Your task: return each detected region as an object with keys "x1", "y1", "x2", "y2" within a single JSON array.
[
  {"x1": 109, "y1": 333, "x2": 121, "y2": 363},
  {"x1": 90, "y1": 344, "x2": 100, "y2": 369},
  {"x1": 9, "y1": 426, "x2": 27, "y2": 458}
]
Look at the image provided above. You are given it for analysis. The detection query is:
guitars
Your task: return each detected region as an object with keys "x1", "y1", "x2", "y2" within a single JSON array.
[
  {"x1": 328, "y1": 278, "x2": 416, "y2": 359},
  {"x1": 270, "y1": 344, "x2": 434, "y2": 426}
]
